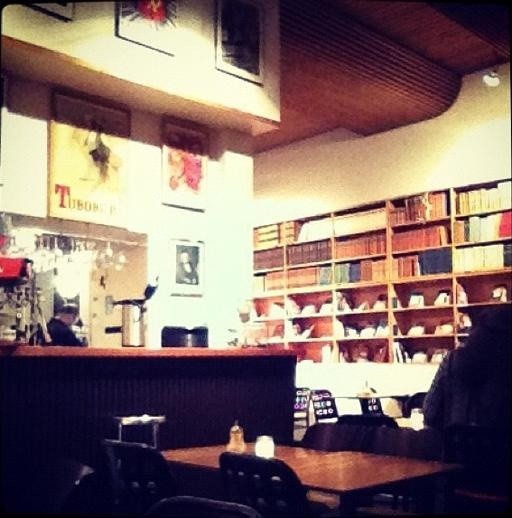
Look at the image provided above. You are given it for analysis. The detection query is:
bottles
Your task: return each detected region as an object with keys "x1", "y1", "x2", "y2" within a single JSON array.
[
  {"x1": 226, "y1": 424, "x2": 246, "y2": 454},
  {"x1": 411, "y1": 408, "x2": 424, "y2": 430},
  {"x1": 255, "y1": 435, "x2": 275, "y2": 459}
]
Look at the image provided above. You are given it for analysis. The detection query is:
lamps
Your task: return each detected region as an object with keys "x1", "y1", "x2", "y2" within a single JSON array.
[{"x1": 482, "y1": 64, "x2": 502, "y2": 87}]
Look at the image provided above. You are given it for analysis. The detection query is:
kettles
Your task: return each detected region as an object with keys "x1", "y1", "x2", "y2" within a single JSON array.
[{"x1": 112, "y1": 276, "x2": 159, "y2": 347}]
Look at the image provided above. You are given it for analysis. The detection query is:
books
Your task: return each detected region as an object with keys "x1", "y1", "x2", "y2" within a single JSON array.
[{"x1": 254, "y1": 181, "x2": 511, "y2": 291}]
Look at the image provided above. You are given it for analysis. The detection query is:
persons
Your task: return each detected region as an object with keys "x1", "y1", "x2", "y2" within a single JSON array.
[
  {"x1": 175, "y1": 252, "x2": 198, "y2": 284},
  {"x1": 42, "y1": 303, "x2": 88, "y2": 346},
  {"x1": 421, "y1": 303, "x2": 512, "y2": 500}
]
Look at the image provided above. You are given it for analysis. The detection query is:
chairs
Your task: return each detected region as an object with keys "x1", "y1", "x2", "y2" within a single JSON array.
[
  {"x1": 110, "y1": 409, "x2": 166, "y2": 461},
  {"x1": 293, "y1": 385, "x2": 313, "y2": 443},
  {"x1": 103, "y1": 434, "x2": 173, "y2": 488},
  {"x1": 214, "y1": 452, "x2": 321, "y2": 513},
  {"x1": 151, "y1": 495, "x2": 258, "y2": 517},
  {"x1": 405, "y1": 391, "x2": 431, "y2": 420},
  {"x1": 359, "y1": 387, "x2": 387, "y2": 416},
  {"x1": 353, "y1": 491, "x2": 445, "y2": 517},
  {"x1": 308, "y1": 390, "x2": 339, "y2": 425}
]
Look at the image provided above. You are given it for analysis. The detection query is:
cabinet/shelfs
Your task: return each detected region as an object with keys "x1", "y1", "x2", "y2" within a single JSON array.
[{"x1": 250, "y1": 178, "x2": 512, "y2": 362}]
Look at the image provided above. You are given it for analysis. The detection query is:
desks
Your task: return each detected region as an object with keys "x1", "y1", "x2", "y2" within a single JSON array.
[
  {"x1": 325, "y1": 392, "x2": 409, "y2": 418},
  {"x1": 155, "y1": 440, "x2": 462, "y2": 517}
]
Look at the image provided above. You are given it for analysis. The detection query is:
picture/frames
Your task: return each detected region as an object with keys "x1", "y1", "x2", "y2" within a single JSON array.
[
  {"x1": 114, "y1": 1, "x2": 181, "y2": 57},
  {"x1": 47, "y1": 88, "x2": 134, "y2": 232},
  {"x1": 160, "y1": 110, "x2": 211, "y2": 214},
  {"x1": 167, "y1": 235, "x2": 206, "y2": 301},
  {"x1": 213, "y1": 0, "x2": 271, "y2": 86},
  {"x1": 25, "y1": 0, "x2": 77, "y2": 24}
]
